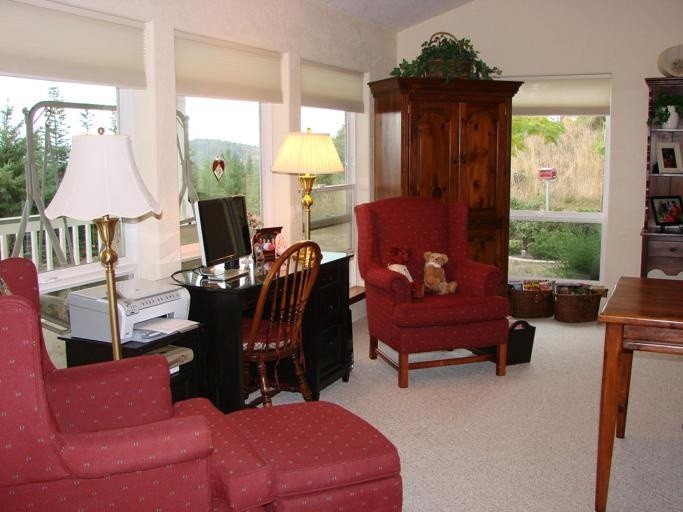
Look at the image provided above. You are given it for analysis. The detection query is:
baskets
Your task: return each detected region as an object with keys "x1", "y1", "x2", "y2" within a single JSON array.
[
  {"x1": 508, "y1": 284, "x2": 555, "y2": 318},
  {"x1": 552, "y1": 290, "x2": 602, "y2": 323}
]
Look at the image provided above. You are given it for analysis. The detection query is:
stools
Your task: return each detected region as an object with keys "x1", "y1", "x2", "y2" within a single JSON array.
[{"x1": 172, "y1": 397, "x2": 403, "y2": 512}]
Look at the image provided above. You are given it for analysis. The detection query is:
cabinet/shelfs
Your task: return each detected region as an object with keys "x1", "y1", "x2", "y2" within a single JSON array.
[
  {"x1": 640, "y1": 76, "x2": 683, "y2": 280},
  {"x1": 367, "y1": 77, "x2": 523, "y2": 299},
  {"x1": 56, "y1": 312, "x2": 219, "y2": 408},
  {"x1": 158, "y1": 252, "x2": 352, "y2": 412}
]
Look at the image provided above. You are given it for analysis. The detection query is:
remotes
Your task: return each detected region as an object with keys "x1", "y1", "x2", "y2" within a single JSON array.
[{"x1": 199, "y1": 266, "x2": 211, "y2": 276}]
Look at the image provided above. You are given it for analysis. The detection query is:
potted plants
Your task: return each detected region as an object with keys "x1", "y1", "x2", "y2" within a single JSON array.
[
  {"x1": 390, "y1": 32, "x2": 500, "y2": 80},
  {"x1": 646, "y1": 93, "x2": 682, "y2": 131}
]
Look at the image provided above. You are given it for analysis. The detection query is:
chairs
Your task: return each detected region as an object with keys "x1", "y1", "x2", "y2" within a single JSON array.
[
  {"x1": 0, "y1": 257, "x2": 211, "y2": 512},
  {"x1": 242, "y1": 241, "x2": 322, "y2": 407},
  {"x1": 353, "y1": 197, "x2": 513, "y2": 387}
]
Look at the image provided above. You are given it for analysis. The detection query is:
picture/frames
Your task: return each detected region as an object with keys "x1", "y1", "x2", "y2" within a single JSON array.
[
  {"x1": 657, "y1": 144, "x2": 683, "y2": 174},
  {"x1": 650, "y1": 195, "x2": 683, "y2": 227}
]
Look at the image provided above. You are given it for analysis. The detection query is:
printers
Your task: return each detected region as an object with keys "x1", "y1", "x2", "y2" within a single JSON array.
[{"x1": 69, "y1": 277, "x2": 200, "y2": 345}]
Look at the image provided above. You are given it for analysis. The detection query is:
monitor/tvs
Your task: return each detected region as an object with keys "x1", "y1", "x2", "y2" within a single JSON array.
[{"x1": 193, "y1": 193, "x2": 253, "y2": 282}]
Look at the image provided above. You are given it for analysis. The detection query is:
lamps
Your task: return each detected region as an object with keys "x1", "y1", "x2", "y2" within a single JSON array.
[
  {"x1": 43, "y1": 128, "x2": 163, "y2": 362},
  {"x1": 271, "y1": 127, "x2": 346, "y2": 259}
]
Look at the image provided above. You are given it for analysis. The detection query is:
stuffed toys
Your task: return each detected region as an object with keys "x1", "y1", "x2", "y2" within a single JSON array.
[
  {"x1": 387, "y1": 245, "x2": 425, "y2": 299},
  {"x1": 425, "y1": 251, "x2": 458, "y2": 297}
]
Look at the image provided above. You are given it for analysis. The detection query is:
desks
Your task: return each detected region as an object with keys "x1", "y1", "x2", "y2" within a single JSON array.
[{"x1": 595, "y1": 276, "x2": 683, "y2": 511}]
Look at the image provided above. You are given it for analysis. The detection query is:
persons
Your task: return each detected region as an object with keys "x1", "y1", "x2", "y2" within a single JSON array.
[
  {"x1": 258, "y1": 237, "x2": 275, "y2": 251},
  {"x1": 657, "y1": 200, "x2": 681, "y2": 221}
]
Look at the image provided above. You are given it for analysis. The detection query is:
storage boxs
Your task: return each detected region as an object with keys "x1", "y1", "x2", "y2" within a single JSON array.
[{"x1": 506, "y1": 317, "x2": 536, "y2": 366}]
